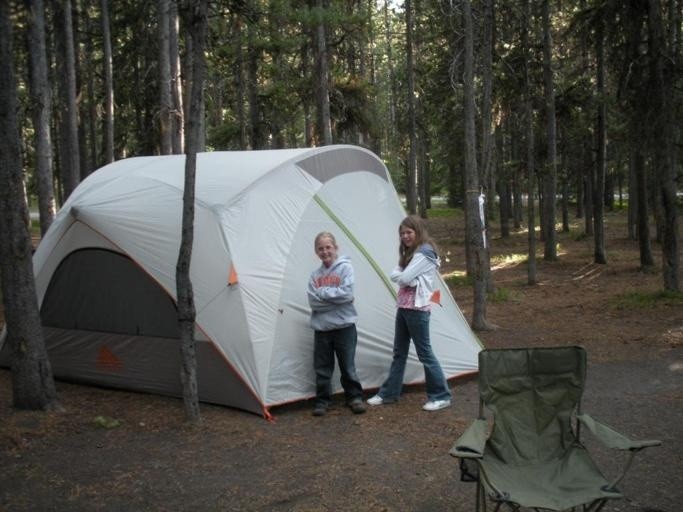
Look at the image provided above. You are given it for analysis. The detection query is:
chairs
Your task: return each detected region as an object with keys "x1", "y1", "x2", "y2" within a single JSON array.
[{"x1": 448, "y1": 345, "x2": 661, "y2": 512}]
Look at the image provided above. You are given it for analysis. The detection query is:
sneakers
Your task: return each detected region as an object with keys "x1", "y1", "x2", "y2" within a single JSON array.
[
  {"x1": 422, "y1": 399, "x2": 450, "y2": 410},
  {"x1": 366, "y1": 393, "x2": 384, "y2": 405},
  {"x1": 312, "y1": 399, "x2": 329, "y2": 416},
  {"x1": 350, "y1": 399, "x2": 365, "y2": 412}
]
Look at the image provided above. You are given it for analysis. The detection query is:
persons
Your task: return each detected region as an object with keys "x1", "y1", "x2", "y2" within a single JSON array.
[
  {"x1": 306, "y1": 231, "x2": 368, "y2": 417},
  {"x1": 367, "y1": 214, "x2": 452, "y2": 412}
]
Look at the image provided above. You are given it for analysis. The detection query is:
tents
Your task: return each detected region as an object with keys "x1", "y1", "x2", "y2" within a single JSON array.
[{"x1": 0, "y1": 143, "x2": 488, "y2": 426}]
